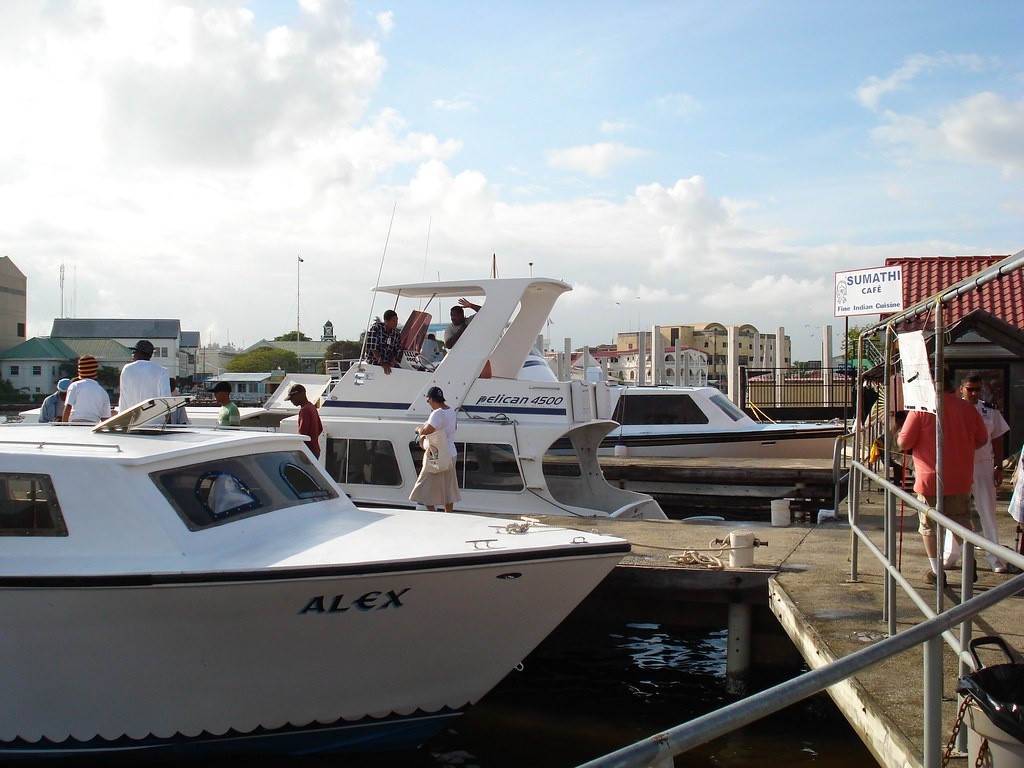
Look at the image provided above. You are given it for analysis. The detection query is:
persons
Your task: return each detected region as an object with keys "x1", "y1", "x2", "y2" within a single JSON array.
[
  {"x1": 118, "y1": 339, "x2": 172, "y2": 416},
  {"x1": 443, "y1": 298, "x2": 481, "y2": 349},
  {"x1": 366, "y1": 310, "x2": 402, "y2": 376},
  {"x1": 942, "y1": 374, "x2": 1006, "y2": 573},
  {"x1": 62, "y1": 354, "x2": 111, "y2": 425},
  {"x1": 338, "y1": 440, "x2": 400, "y2": 487},
  {"x1": 169, "y1": 378, "x2": 189, "y2": 427},
  {"x1": 39, "y1": 378, "x2": 72, "y2": 424},
  {"x1": 288, "y1": 384, "x2": 322, "y2": 461},
  {"x1": 954, "y1": 370, "x2": 1005, "y2": 416},
  {"x1": 415, "y1": 386, "x2": 458, "y2": 513},
  {"x1": 213, "y1": 382, "x2": 240, "y2": 432},
  {"x1": 891, "y1": 411, "x2": 916, "y2": 493},
  {"x1": 897, "y1": 367, "x2": 990, "y2": 592},
  {"x1": 419, "y1": 334, "x2": 440, "y2": 363}
]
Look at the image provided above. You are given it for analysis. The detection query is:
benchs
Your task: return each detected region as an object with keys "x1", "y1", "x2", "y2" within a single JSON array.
[{"x1": 877, "y1": 431, "x2": 916, "y2": 494}]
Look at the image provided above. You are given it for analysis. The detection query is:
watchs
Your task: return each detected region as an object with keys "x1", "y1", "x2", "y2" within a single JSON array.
[{"x1": 996, "y1": 465, "x2": 1002, "y2": 471}]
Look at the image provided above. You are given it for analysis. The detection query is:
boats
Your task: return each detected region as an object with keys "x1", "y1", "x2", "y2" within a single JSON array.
[
  {"x1": 367, "y1": 343, "x2": 857, "y2": 506},
  {"x1": 1, "y1": 273, "x2": 670, "y2": 523},
  {"x1": 1, "y1": 397, "x2": 633, "y2": 768}
]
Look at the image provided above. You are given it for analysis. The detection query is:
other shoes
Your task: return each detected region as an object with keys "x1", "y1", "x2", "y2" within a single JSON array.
[
  {"x1": 973, "y1": 559, "x2": 979, "y2": 583},
  {"x1": 987, "y1": 560, "x2": 1005, "y2": 572},
  {"x1": 942, "y1": 559, "x2": 952, "y2": 570},
  {"x1": 921, "y1": 569, "x2": 948, "y2": 588}
]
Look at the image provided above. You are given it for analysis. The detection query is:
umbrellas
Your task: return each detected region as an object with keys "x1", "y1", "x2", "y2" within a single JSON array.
[{"x1": 852, "y1": 378, "x2": 880, "y2": 426}]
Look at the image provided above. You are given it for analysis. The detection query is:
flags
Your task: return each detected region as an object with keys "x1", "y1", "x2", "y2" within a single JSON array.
[{"x1": 298, "y1": 257, "x2": 303, "y2": 263}]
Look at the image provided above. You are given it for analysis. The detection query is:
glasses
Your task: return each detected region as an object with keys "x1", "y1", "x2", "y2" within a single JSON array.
[
  {"x1": 132, "y1": 350, "x2": 137, "y2": 354},
  {"x1": 427, "y1": 398, "x2": 430, "y2": 403},
  {"x1": 964, "y1": 387, "x2": 982, "y2": 392}
]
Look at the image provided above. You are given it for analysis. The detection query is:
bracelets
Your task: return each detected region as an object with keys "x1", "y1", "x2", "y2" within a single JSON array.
[{"x1": 377, "y1": 356, "x2": 381, "y2": 364}]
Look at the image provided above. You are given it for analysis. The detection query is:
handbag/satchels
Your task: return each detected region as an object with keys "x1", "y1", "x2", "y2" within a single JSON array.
[{"x1": 420, "y1": 408, "x2": 453, "y2": 474}]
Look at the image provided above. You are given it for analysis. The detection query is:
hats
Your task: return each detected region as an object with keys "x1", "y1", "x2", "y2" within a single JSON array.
[
  {"x1": 284, "y1": 384, "x2": 304, "y2": 401},
  {"x1": 207, "y1": 382, "x2": 231, "y2": 393},
  {"x1": 427, "y1": 386, "x2": 445, "y2": 402},
  {"x1": 78, "y1": 356, "x2": 99, "y2": 379},
  {"x1": 128, "y1": 340, "x2": 154, "y2": 354},
  {"x1": 57, "y1": 378, "x2": 73, "y2": 393}
]
